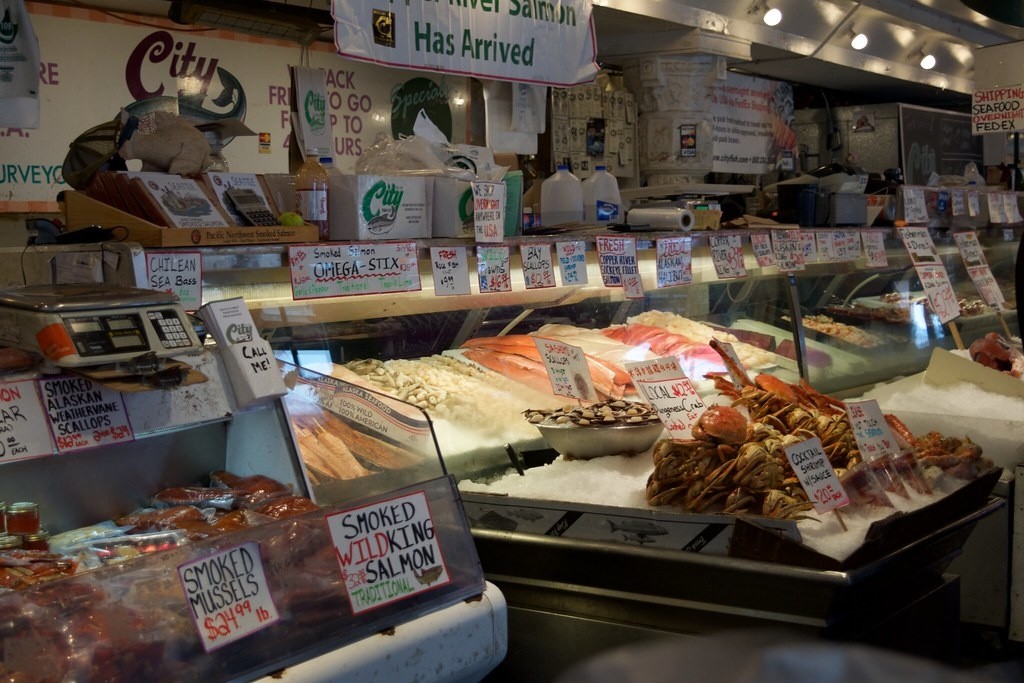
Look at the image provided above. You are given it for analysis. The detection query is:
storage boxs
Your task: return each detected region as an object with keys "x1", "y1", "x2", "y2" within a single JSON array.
[
  {"x1": 63, "y1": 170, "x2": 524, "y2": 248},
  {"x1": 688, "y1": 208, "x2": 723, "y2": 231}
]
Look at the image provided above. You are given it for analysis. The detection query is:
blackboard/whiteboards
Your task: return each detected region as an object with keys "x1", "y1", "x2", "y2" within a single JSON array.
[{"x1": 898, "y1": 103, "x2": 984, "y2": 187}]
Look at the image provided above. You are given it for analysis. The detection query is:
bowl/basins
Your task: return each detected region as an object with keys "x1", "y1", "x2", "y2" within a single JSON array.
[{"x1": 537, "y1": 424, "x2": 665, "y2": 458}]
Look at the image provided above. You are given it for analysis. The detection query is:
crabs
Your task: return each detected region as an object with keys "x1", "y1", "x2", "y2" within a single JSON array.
[{"x1": 645, "y1": 339, "x2": 996, "y2": 525}]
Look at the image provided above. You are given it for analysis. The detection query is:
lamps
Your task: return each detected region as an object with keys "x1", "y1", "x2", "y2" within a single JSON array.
[
  {"x1": 837, "y1": 21, "x2": 868, "y2": 50},
  {"x1": 907, "y1": 41, "x2": 936, "y2": 70},
  {"x1": 745, "y1": 0, "x2": 782, "y2": 26}
]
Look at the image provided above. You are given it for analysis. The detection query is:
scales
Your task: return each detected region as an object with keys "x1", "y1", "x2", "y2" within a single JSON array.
[
  {"x1": 0, "y1": 281, "x2": 203, "y2": 368},
  {"x1": 619, "y1": 184, "x2": 760, "y2": 222}
]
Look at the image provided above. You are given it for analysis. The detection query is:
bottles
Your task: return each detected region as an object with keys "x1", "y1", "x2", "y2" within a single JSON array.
[
  {"x1": 296, "y1": 149, "x2": 339, "y2": 242},
  {"x1": 541, "y1": 165, "x2": 596, "y2": 225},
  {"x1": 582, "y1": 166, "x2": 624, "y2": 226}
]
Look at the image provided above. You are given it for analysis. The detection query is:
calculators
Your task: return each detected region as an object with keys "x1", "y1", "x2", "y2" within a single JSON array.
[{"x1": 226, "y1": 188, "x2": 281, "y2": 227}]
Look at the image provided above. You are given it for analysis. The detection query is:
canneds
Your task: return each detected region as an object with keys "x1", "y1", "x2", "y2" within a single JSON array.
[{"x1": 0, "y1": 502, "x2": 50, "y2": 552}]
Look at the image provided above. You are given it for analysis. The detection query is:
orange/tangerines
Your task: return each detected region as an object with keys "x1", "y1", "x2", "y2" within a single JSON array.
[{"x1": 279, "y1": 212, "x2": 304, "y2": 227}]
